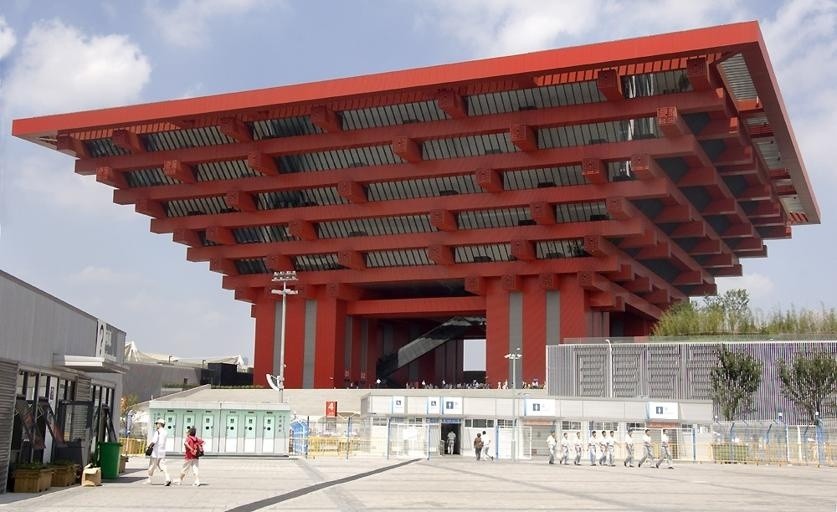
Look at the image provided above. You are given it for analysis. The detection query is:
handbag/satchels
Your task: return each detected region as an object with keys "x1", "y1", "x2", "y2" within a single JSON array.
[{"x1": 147, "y1": 443, "x2": 154, "y2": 456}]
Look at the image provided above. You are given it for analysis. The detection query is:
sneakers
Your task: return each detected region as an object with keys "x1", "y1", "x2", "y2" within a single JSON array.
[{"x1": 164, "y1": 481, "x2": 171, "y2": 486}]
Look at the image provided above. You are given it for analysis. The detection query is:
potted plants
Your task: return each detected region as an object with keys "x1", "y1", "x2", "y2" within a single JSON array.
[
  {"x1": 11, "y1": 461, "x2": 51, "y2": 494},
  {"x1": 46, "y1": 459, "x2": 80, "y2": 488}
]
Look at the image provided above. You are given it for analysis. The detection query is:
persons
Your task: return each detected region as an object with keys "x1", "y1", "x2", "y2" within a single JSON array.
[
  {"x1": 637, "y1": 429, "x2": 655, "y2": 468},
  {"x1": 655, "y1": 429, "x2": 673, "y2": 469},
  {"x1": 605, "y1": 430, "x2": 621, "y2": 467},
  {"x1": 585, "y1": 430, "x2": 600, "y2": 465},
  {"x1": 559, "y1": 432, "x2": 572, "y2": 465},
  {"x1": 573, "y1": 431, "x2": 584, "y2": 465},
  {"x1": 545, "y1": 430, "x2": 557, "y2": 464},
  {"x1": 623, "y1": 429, "x2": 634, "y2": 467},
  {"x1": 481, "y1": 430, "x2": 494, "y2": 461},
  {"x1": 598, "y1": 431, "x2": 607, "y2": 465},
  {"x1": 447, "y1": 429, "x2": 456, "y2": 456},
  {"x1": 376, "y1": 377, "x2": 537, "y2": 389},
  {"x1": 473, "y1": 433, "x2": 483, "y2": 459},
  {"x1": 178, "y1": 427, "x2": 206, "y2": 486},
  {"x1": 143, "y1": 418, "x2": 172, "y2": 487}
]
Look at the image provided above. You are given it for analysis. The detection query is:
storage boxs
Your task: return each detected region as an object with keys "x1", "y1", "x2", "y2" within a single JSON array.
[{"x1": 79, "y1": 465, "x2": 103, "y2": 488}]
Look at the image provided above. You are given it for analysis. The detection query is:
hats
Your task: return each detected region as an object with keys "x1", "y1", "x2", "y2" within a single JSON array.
[{"x1": 154, "y1": 419, "x2": 166, "y2": 426}]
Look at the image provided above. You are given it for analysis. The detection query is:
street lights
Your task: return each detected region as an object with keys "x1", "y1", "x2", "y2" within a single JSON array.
[
  {"x1": 270, "y1": 270, "x2": 298, "y2": 403},
  {"x1": 503, "y1": 348, "x2": 522, "y2": 463}
]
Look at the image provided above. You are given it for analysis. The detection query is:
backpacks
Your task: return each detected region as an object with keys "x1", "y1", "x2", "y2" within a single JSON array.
[{"x1": 189, "y1": 437, "x2": 204, "y2": 457}]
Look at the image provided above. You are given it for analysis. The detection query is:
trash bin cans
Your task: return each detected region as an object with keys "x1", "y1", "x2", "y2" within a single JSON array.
[{"x1": 98, "y1": 443, "x2": 123, "y2": 480}]
